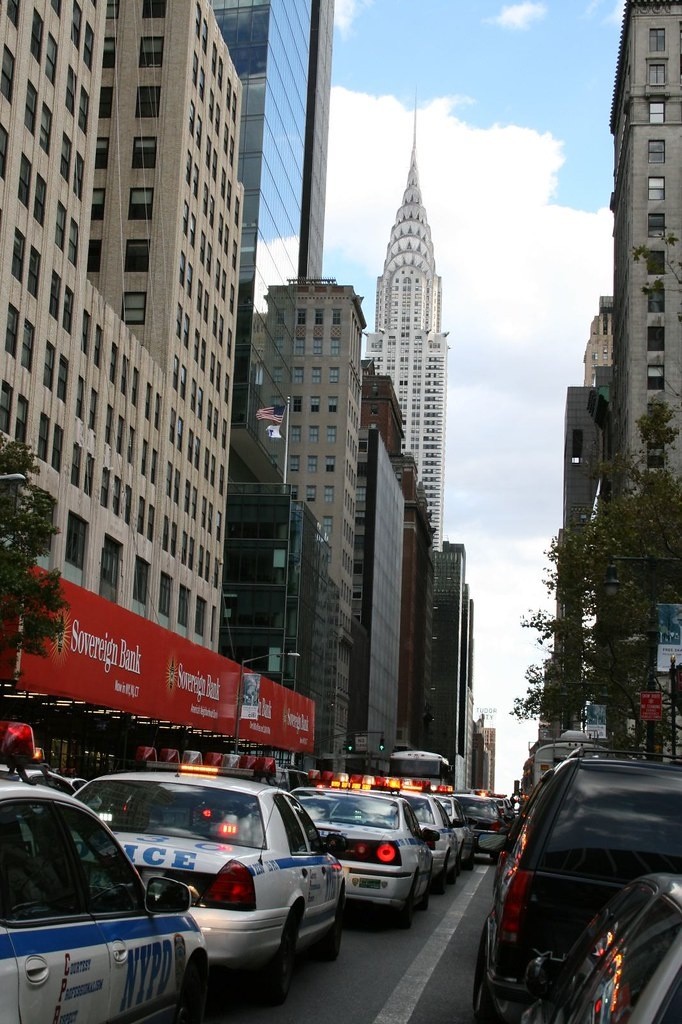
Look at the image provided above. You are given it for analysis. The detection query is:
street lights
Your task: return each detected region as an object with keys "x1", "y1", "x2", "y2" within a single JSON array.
[{"x1": 232, "y1": 652, "x2": 300, "y2": 754}]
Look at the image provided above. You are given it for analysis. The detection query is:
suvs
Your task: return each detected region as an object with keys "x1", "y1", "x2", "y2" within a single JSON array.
[{"x1": 469, "y1": 748, "x2": 682, "y2": 1024}]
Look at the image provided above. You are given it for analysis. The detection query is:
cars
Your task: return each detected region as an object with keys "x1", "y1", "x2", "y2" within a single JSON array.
[
  {"x1": 0, "y1": 718, "x2": 209, "y2": 1024},
  {"x1": 518, "y1": 873, "x2": 682, "y2": 1023},
  {"x1": 283, "y1": 768, "x2": 517, "y2": 928},
  {"x1": 0, "y1": 747, "x2": 103, "y2": 823},
  {"x1": 63, "y1": 746, "x2": 346, "y2": 1007}
]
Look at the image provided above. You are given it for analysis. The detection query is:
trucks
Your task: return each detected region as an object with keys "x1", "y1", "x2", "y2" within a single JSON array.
[{"x1": 519, "y1": 731, "x2": 615, "y2": 809}]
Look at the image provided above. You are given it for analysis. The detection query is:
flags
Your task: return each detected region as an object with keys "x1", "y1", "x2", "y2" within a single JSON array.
[
  {"x1": 586, "y1": 706, "x2": 606, "y2": 739},
  {"x1": 657, "y1": 604, "x2": 682, "y2": 672}
]
